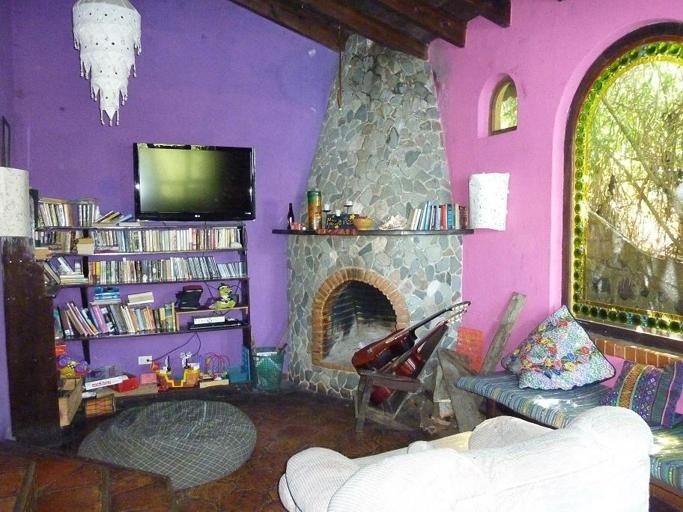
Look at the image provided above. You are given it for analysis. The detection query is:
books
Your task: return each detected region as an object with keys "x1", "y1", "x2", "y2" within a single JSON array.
[
  {"x1": 81, "y1": 367, "x2": 129, "y2": 398},
  {"x1": 187, "y1": 315, "x2": 244, "y2": 330},
  {"x1": 406, "y1": 202, "x2": 470, "y2": 231},
  {"x1": 53, "y1": 287, "x2": 181, "y2": 340},
  {"x1": 35, "y1": 199, "x2": 246, "y2": 285},
  {"x1": 199, "y1": 379, "x2": 229, "y2": 388}
]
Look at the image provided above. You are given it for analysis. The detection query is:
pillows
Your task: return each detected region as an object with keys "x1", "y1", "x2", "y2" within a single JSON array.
[
  {"x1": 502, "y1": 307, "x2": 615, "y2": 391},
  {"x1": 599, "y1": 360, "x2": 683, "y2": 430}
]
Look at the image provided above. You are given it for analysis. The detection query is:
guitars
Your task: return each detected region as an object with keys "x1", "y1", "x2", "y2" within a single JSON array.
[{"x1": 351, "y1": 300, "x2": 470, "y2": 404}]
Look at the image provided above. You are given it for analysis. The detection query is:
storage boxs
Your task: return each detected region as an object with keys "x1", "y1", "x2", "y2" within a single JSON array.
[
  {"x1": 106, "y1": 371, "x2": 140, "y2": 392},
  {"x1": 82, "y1": 393, "x2": 116, "y2": 419},
  {"x1": 59, "y1": 378, "x2": 82, "y2": 427}
]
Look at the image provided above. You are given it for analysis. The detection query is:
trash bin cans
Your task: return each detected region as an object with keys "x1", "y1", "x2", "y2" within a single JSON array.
[{"x1": 252, "y1": 347, "x2": 286, "y2": 392}]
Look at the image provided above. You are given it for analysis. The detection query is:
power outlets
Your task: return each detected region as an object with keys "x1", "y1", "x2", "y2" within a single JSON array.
[{"x1": 138, "y1": 356, "x2": 153, "y2": 365}]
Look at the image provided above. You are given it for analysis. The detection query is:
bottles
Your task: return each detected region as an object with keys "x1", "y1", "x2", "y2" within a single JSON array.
[
  {"x1": 321, "y1": 204, "x2": 332, "y2": 229},
  {"x1": 287, "y1": 203, "x2": 296, "y2": 230},
  {"x1": 344, "y1": 202, "x2": 353, "y2": 214}
]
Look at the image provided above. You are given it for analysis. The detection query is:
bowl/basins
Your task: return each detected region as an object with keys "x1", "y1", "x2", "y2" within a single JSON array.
[{"x1": 353, "y1": 217, "x2": 374, "y2": 230}]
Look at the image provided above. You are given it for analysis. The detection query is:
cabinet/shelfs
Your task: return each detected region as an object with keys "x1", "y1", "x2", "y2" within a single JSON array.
[
  {"x1": 29, "y1": 188, "x2": 256, "y2": 422},
  {"x1": 3, "y1": 263, "x2": 60, "y2": 438}
]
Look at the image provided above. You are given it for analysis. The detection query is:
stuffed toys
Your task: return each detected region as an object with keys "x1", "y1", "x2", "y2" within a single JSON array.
[{"x1": 210, "y1": 283, "x2": 236, "y2": 311}]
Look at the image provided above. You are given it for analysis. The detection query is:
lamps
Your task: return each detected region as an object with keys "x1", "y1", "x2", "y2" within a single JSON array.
[{"x1": 1, "y1": 166, "x2": 29, "y2": 265}]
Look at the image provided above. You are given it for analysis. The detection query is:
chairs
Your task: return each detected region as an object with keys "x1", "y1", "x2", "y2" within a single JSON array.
[{"x1": 278, "y1": 407, "x2": 651, "y2": 512}]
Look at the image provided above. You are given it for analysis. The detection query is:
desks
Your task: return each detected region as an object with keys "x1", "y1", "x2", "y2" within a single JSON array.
[{"x1": 0, "y1": 438, "x2": 171, "y2": 511}]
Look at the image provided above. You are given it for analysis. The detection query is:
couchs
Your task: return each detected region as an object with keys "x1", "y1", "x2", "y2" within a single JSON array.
[{"x1": 456, "y1": 335, "x2": 683, "y2": 512}]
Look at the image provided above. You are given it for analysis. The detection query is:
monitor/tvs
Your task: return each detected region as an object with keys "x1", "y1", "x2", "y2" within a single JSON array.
[{"x1": 133, "y1": 142, "x2": 256, "y2": 221}]
